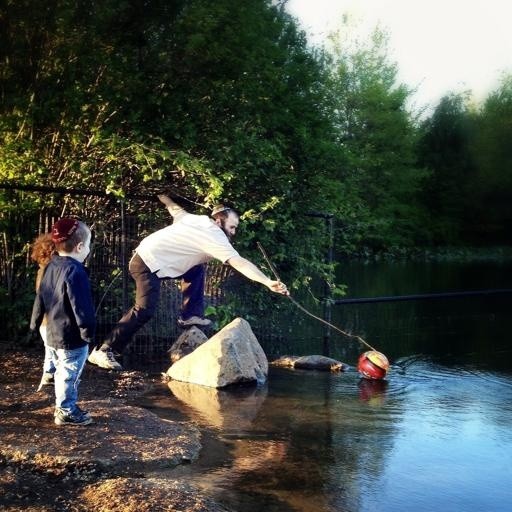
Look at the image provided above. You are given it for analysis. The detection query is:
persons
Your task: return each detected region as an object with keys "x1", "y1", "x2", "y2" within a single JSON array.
[
  {"x1": 32, "y1": 232, "x2": 57, "y2": 387},
  {"x1": 31, "y1": 218, "x2": 97, "y2": 426},
  {"x1": 87, "y1": 196, "x2": 291, "y2": 368}
]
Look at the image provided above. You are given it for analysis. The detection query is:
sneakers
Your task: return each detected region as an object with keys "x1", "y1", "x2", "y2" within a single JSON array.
[
  {"x1": 87, "y1": 345, "x2": 122, "y2": 370},
  {"x1": 54, "y1": 408, "x2": 93, "y2": 426},
  {"x1": 176, "y1": 315, "x2": 212, "y2": 328}
]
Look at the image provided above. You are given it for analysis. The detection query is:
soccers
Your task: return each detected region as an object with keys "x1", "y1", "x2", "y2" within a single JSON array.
[{"x1": 357, "y1": 350, "x2": 389, "y2": 379}]
